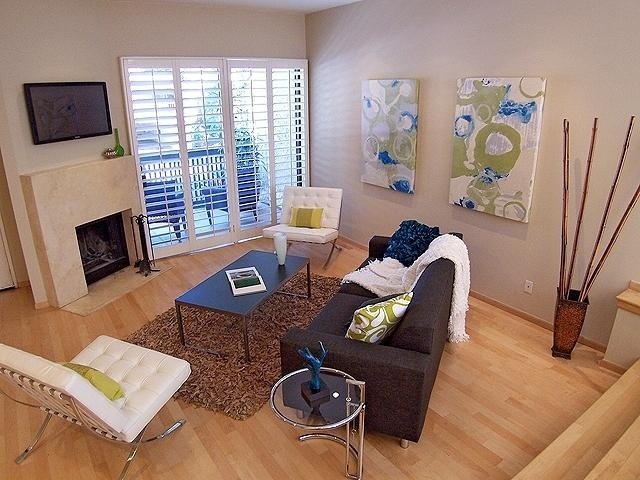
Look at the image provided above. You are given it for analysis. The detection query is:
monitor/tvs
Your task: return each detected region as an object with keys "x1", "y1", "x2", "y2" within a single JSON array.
[{"x1": 22, "y1": 81, "x2": 113, "y2": 145}]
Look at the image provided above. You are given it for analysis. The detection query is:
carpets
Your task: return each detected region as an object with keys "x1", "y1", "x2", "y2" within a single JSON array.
[{"x1": 119, "y1": 272, "x2": 342, "y2": 423}]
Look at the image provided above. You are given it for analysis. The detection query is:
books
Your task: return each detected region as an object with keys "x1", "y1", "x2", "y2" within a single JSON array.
[{"x1": 225, "y1": 266, "x2": 267, "y2": 297}]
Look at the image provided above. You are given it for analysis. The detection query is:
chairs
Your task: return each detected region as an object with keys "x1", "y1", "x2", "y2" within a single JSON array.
[
  {"x1": 141, "y1": 171, "x2": 184, "y2": 244},
  {"x1": 202, "y1": 168, "x2": 260, "y2": 227},
  {"x1": 0, "y1": 333, "x2": 191, "y2": 480}
]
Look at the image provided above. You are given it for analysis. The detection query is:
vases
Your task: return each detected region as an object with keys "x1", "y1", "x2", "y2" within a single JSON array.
[
  {"x1": 550, "y1": 288, "x2": 589, "y2": 358},
  {"x1": 114, "y1": 127, "x2": 124, "y2": 157}
]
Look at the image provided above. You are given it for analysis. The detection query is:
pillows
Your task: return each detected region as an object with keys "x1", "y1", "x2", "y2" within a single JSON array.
[
  {"x1": 288, "y1": 207, "x2": 325, "y2": 229},
  {"x1": 341, "y1": 290, "x2": 414, "y2": 342},
  {"x1": 346, "y1": 293, "x2": 400, "y2": 331},
  {"x1": 57, "y1": 361, "x2": 126, "y2": 400}
]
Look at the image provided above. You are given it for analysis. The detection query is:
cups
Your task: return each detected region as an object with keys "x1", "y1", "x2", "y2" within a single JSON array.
[{"x1": 273, "y1": 232, "x2": 287, "y2": 266}]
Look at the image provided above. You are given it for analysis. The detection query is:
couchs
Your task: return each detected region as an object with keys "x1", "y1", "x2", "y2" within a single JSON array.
[
  {"x1": 262, "y1": 184, "x2": 342, "y2": 272},
  {"x1": 279, "y1": 218, "x2": 470, "y2": 449}
]
